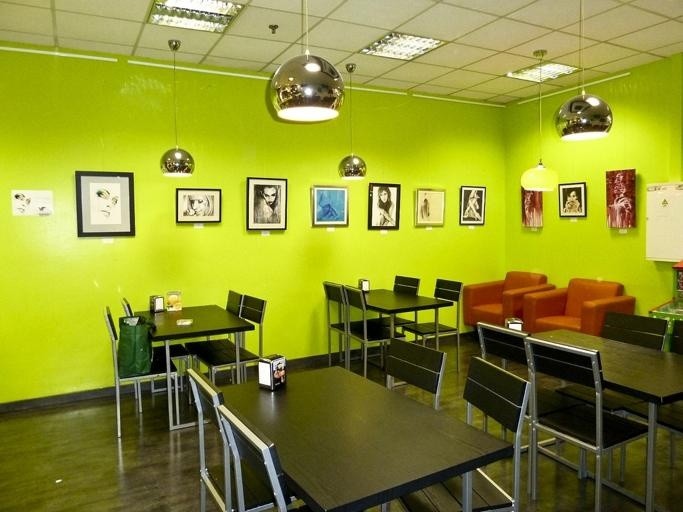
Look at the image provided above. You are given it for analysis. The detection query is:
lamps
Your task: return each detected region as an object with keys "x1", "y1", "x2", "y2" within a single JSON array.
[
  {"x1": 269, "y1": 0, "x2": 344, "y2": 122},
  {"x1": 553, "y1": 0, "x2": 612, "y2": 139},
  {"x1": 160, "y1": 39, "x2": 195, "y2": 176},
  {"x1": 337, "y1": 63, "x2": 366, "y2": 179},
  {"x1": 520, "y1": 50, "x2": 558, "y2": 192}
]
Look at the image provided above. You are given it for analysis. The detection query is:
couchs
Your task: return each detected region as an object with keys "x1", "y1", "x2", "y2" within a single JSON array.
[
  {"x1": 461, "y1": 271, "x2": 555, "y2": 341},
  {"x1": 521, "y1": 278, "x2": 635, "y2": 337}
]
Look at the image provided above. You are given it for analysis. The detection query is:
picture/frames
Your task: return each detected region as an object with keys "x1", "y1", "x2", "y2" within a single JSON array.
[
  {"x1": 520, "y1": 186, "x2": 543, "y2": 228},
  {"x1": 246, "y1": 177, "x2": 287, "y2": 230},
  {"x1": 175, "y1": 188, "x2": 221, "y2": 223},
  {"x1": 414, "y1": 188, "x2": 445, "y2": 227},
  {"x1": 557, "y1": 181, "x2": 586, "y2": 217},
  {"x1": 311, "y1": 185, "x2": 348, "y2": 227},
  {"x1": 367, "y1": 182, "x2": 400, "y2": 230},
  {"x1": 458, "y1": 186, "x2": 485, "y2": 225},
  {"x1": 604, "y1": 169, "x2": 636, "y2": 229},
  {"x1": 75, "y1": 170, "x2": 135, "y2": 238}
]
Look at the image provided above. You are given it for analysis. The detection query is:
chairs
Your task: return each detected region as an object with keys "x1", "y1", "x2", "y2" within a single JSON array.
[
  {"x1": 380, "y1": 357, "x2": 531, "y2": 512},
  {"x1": 184, "y1": 290, "x2": 243, "y2": 385},
  {"x1": 194, "y1": 294, "x2": 267, "y2": 405},
  {"x1": 217, "y1": 403, "x2": 321, "y2": 512},
  {"x1": 401, "y1": 279, "x2": 463, "y2": 371},
  {"x1": 322, "y1": 281, "x2": 385, "y2": 370},
  {"x1": 366, "y1": 275, "x2": 420, "y2": 345},
  {"x1": 341, "y1": 284, "x2": 405, "y2": 380},
  {"x1": 120, "y1": 297, "x2": 191, "y2": 414},
  {"x1": 475, "y1": 322, "x2": 587, "y2": 496},
  {"x1": 382, "y1": 338, "x2": 447, "y2": 412},
  {"x1": 525, "y1": 336, "x2": 648, "y2": 512},
  {"x1": 619, "y1": 320, "x2": 682, "y2": 473},
  {"x1": 186, "y1": 367, "x2": 297, "y2": 512},
  {"x1": 102, "y1": 306, "x2": 179, "y2": 438},
  {"x1": 549, "y1": 310, "x2": 668, "y2": 464}
]
